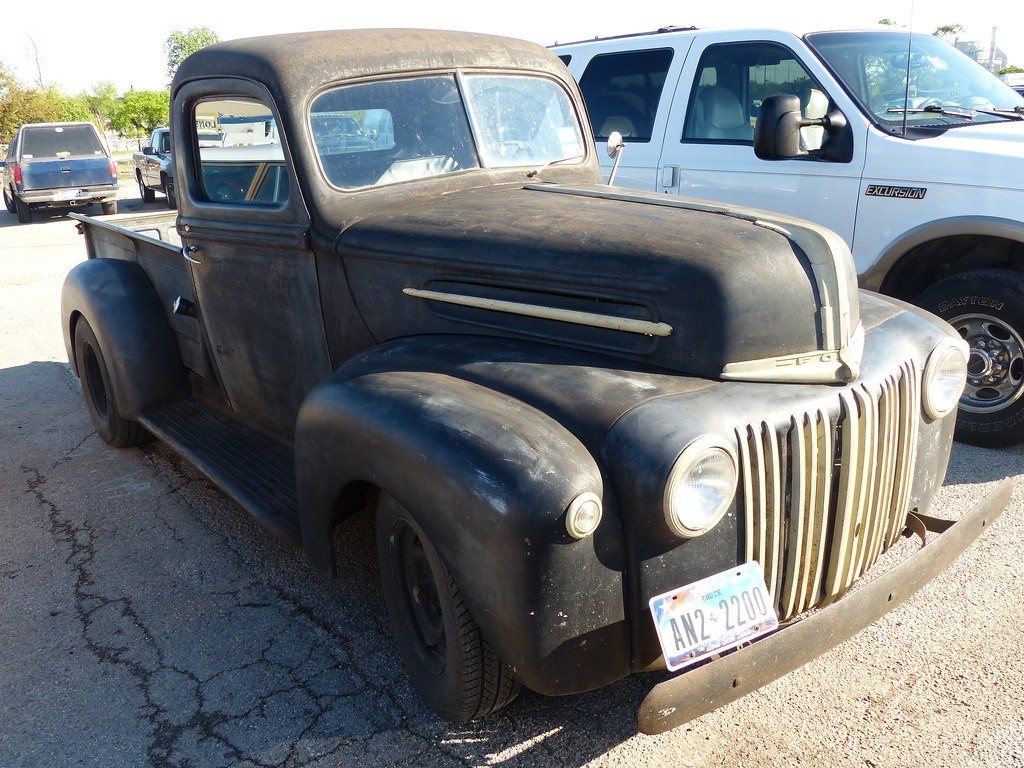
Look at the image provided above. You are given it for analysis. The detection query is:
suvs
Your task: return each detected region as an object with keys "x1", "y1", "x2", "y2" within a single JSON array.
[
  {"x1": 519, "y1": 23, "x2": 1024, "y2": 454},
  {"x1": 269, "y1": 111, "x2": 378, "y2": 156}
]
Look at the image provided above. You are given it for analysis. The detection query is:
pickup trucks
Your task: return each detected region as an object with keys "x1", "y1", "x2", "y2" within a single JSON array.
[{"x1": 131, "y1": 128, "x2": 221, "y2": 209}]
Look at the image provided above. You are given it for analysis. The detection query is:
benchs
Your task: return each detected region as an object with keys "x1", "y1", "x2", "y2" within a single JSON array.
[
  {"x1": 592, "y1": 91, "x2": 660, "y2": 140},
  {"x1": 333, "y1": 154, "x2": 460, "y2": 186}
]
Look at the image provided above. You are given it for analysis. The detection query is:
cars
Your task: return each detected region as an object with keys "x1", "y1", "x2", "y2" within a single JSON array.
[
  {"x1": 59, "y1": 26, "x2": 1013, "y2": 740},
  {"x1": 0, "y1": 119, "x2": 119, "y2": 223}
]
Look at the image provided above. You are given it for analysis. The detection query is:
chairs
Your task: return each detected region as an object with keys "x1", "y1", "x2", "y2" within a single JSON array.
[
  {"x1": 797, "y1": 89, "x2": 828, "y2": 149},
  {"x1": 693, "y1": 87, "x2": 756, "y2": 142}
]
